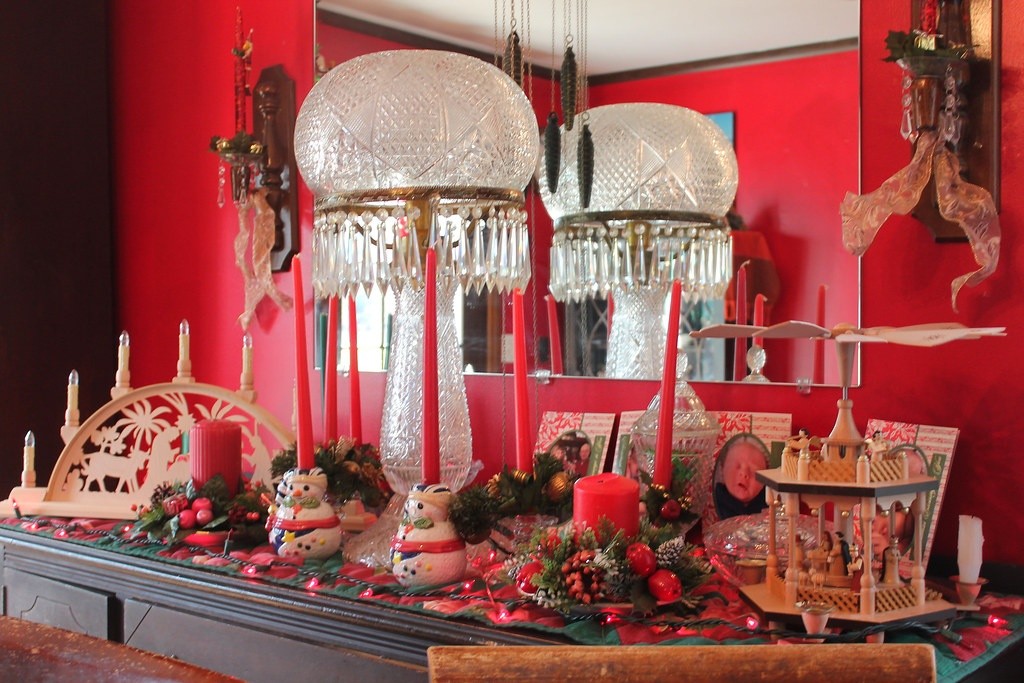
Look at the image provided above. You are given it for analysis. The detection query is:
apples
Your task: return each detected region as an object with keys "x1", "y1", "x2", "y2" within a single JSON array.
[
  {"x1": 178, "y1": 497, "x2": 213, "y2": 529},
  {"x1": 515, "y1": 561, "x2": 545, "y2": 593},
  {"x1": 627, "y1": 543, "x2": 681, "y2": 601}
]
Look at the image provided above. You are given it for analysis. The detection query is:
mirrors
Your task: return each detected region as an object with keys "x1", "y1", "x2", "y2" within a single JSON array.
[{"x1": 314, "y1": 0, "x2": 860, "y2": 390}]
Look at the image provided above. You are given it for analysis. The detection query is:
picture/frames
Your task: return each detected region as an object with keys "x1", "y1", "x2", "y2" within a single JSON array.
[
  {"x1": 613, "y1": 411, "x2": 655, "y2": 521},
  {"x1": 689, "y1": 412, "x2": 792, "y2": 534},
  {"x1": 531, "y1": 411, "x2": 616, "y2": 479},
  {"x1": 853, "y1": 419, "x2": 962, "y2": 581}
]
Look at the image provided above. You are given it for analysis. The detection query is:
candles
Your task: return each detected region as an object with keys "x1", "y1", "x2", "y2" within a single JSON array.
[
  {"x1": 733, "y1": 259, "x2": 750, "y2": 383},
  {"x1": 812, "y1": 283, "x2": 825, "y2": 386},
  {"x1": 653, "y1": 274, "x2": 681, "y2": 491},
  {"x1": 291, "y1": 252, "x2": 316, "y2": 472},
  {"x1": 188, "y1": 418, "x2": 242, "y2": 502},
  {"x1": 606, "y1": 288, "x2": 614, "y2": 342},
  {"x1": 322, "y1": 292, "x2": 339, "y2": 450},
  {"x1": 572, "y1": 472, "x2": 639, "y2": 548},
  {"x1": 956, "y1": 510, "x2": 987, "y2": 585},
  {"x1": 420, "y1": 240, "x2": 443, "y2": 491},
  {"x1": 541, "y1": 292, "x2": 563, "y2": 375},
  {"x1": 512, "y1": 286, "x2": 534, "y2": 477},
  {"x1": 752, "y1": 292, "x2": 767, "y2": 375},
  {"x1": 349, "y1": 290, "x2": 364, "y2": 448}
]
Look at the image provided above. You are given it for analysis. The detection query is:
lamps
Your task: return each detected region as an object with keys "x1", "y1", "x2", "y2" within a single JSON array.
[
  {"x1": 534, "y1": 103, "x2": 739, "y2": 380},
  {"x1": 292, "y1": 47, "x2": 542, "y2": 570}
]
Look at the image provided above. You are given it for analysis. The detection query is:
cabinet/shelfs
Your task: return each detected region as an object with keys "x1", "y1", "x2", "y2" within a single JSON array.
[{"x1": 0, "y1": 514, "x2": 1022, "y2": 683}]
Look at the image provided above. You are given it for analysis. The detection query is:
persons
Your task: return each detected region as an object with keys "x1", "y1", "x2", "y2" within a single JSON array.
[
  {"x1": 716, "y1": 441, "x2": 769, "y2": 520},
  {"x1": 787, "y1": 428, "x2": 810, "y2": 458},
  {"x1": 549, "y1": 430, "x2": 591, "y2": 477},
  {"x1": 868, "y1": 430, "x2": 887, "y2": 464},
  {"x1": 797, "y1": 530, "x2": 905, "y2": 587}
]
